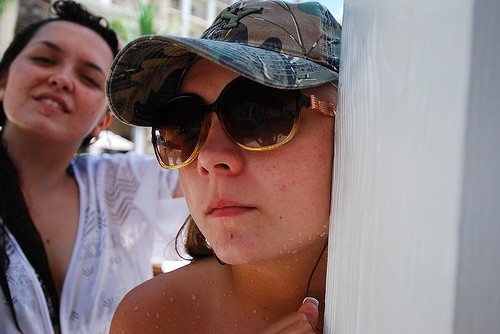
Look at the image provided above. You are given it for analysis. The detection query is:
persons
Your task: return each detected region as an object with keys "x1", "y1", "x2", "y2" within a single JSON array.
[
  {"x1": 108, "y1": 1, "x2": 344, "y2": 334},
  {"x1": 1, "y1": 0, "x2": 189, "y2": 334}
]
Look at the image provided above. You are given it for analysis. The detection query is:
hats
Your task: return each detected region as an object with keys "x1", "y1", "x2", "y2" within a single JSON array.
[{"x1": 103, "y1": 0, "x2": 343, "y2": 126}]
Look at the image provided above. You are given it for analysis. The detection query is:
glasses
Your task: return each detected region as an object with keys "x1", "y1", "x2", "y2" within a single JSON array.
[{"x1": 150, "y1": 73, "x2": 339, "y2": 169}]
